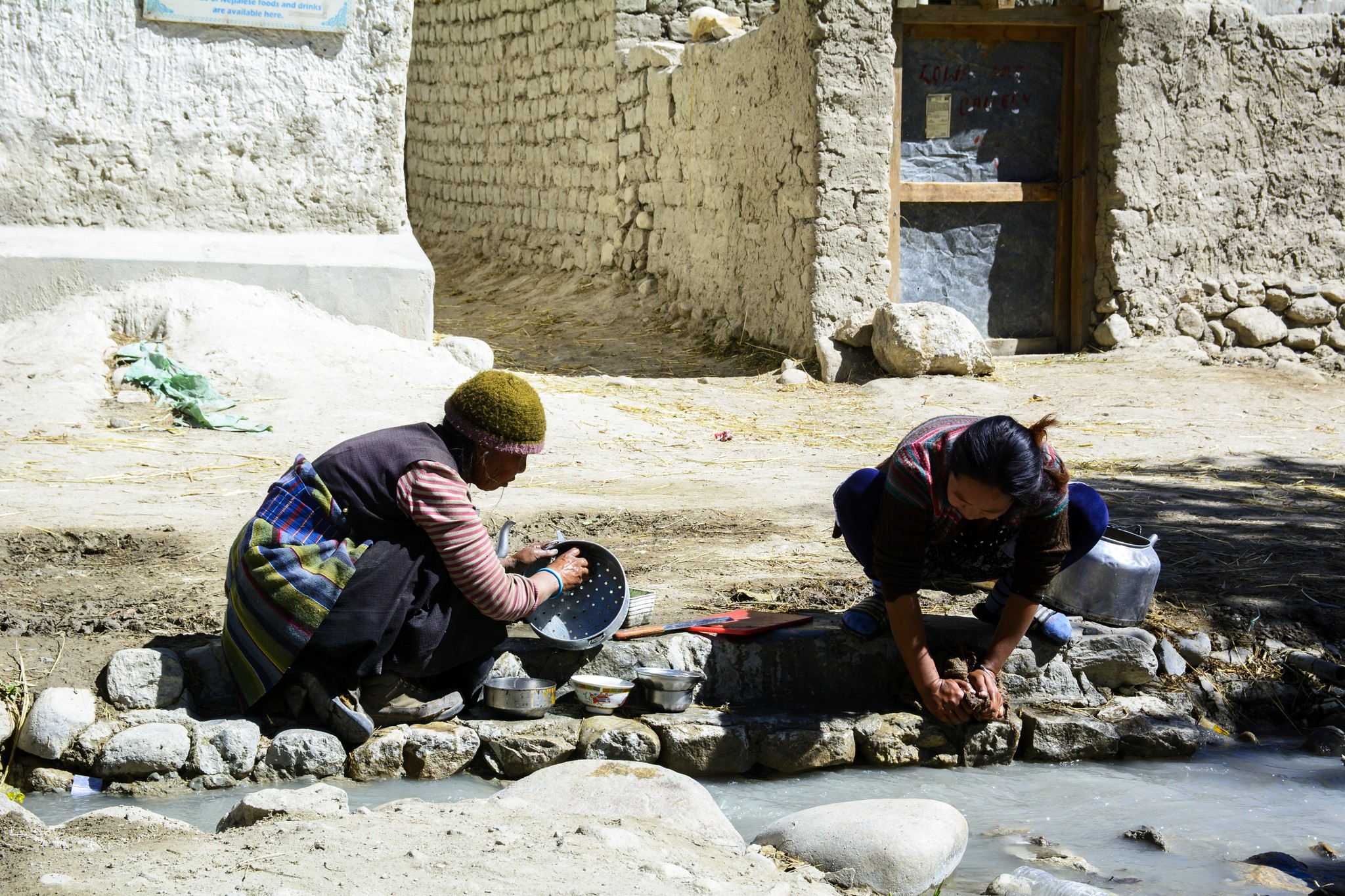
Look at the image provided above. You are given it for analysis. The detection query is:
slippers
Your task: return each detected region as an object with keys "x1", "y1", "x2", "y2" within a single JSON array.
[
  {"x1": 971, "y1": 602, "x2": 1071, "y2": 646},
  {"x1": 839, "y1": 596, "x2": 922, "y2": 640}
]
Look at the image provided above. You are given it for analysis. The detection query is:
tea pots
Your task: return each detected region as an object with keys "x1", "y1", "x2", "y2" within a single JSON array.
[
  {"x1": 476, "y1": 509, "x2": 518, "y2": 558},
  {"x1": 1041, "y1": 522, "x2": 1161, "y2": 627}
]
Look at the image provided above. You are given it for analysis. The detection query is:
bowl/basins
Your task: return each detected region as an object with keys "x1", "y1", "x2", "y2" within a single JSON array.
[
  {"x1": 633, "y1": 667, "x2": 705, "y2": 691},
  {"x1": 483, "y1": 677, "x2": 557, "y2": 718},
  {"x1": 621, "y1": 586, "x2": 656, "y2": 628},
  {"x1": 571, "y1": 675, "x2": 635, "y2": 714},
  {"x1": 643, "y1": 685, "x2": 694, "y2": 713}
]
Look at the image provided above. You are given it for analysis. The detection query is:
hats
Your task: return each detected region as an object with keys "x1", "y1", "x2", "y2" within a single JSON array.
[{"x1": 444, "y1": 369, "x2": 546, "y2": 455}]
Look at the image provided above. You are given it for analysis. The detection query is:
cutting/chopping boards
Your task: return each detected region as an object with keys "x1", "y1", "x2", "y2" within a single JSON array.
[{"x1": 679, "y1": 609, "x2": 813, "y2": 635}]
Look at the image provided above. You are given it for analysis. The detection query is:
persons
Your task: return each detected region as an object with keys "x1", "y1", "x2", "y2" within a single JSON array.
[
  {"x1": 832, "y1": 413, "x2": 1109, "y2": 724},
  {"x1": 221, "y1": 369, "x2": 589, "y2": 745}
]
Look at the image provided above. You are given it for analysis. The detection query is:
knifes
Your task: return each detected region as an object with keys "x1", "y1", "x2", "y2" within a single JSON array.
[{"x1": 612, "y1": 616, "x2": 735, "y2": 641}]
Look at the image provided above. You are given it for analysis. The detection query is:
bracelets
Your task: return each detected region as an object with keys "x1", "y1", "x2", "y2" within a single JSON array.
[
  {"x1": 537, "y1": 568, "x2": 564, "y2": 599},
  {"x1": 979, "y1": 664, "x2": 997, "y2": 685}
]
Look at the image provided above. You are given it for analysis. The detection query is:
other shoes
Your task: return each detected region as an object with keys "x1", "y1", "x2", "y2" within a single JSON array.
[
  {"x1": 367, "y1": 670, "x2": 466, "y2": 730},
  {"x1": 303, "y1": 670, "x2": 374, "y2": 746}
]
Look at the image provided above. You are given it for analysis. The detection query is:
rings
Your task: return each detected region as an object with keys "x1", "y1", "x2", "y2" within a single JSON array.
[{"x1": 997, "y1": 709, "x2": 1000, "y2": 712}]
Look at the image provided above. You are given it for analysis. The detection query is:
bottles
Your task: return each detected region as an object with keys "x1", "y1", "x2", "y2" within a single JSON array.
[{"x1": 1006, "y1": 865, "x2": 1109, "y2": 896}]
